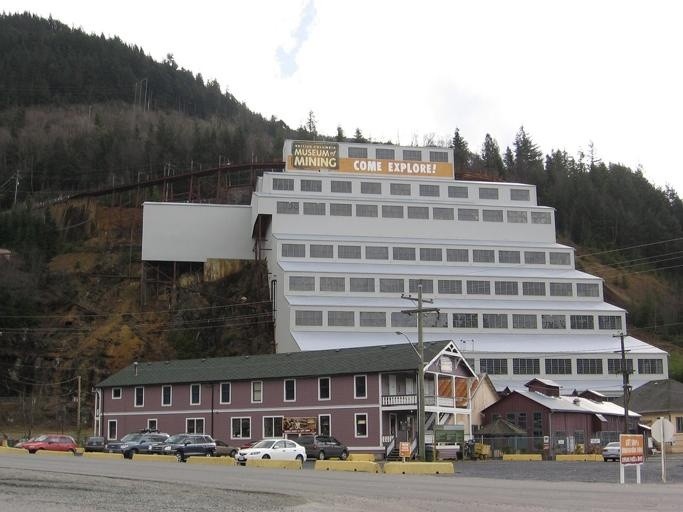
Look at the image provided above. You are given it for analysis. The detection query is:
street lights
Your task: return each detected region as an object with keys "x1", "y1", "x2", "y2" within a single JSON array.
[{"x1": 396, "y1": 282, "x2": 439, "y2": 462}]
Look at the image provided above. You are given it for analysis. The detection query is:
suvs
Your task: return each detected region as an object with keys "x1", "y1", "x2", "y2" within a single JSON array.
[
  {"x1": 104, "y1": 427, "x2": 169, "y2": 454},
  {"x1": 150, "y1": 433, "x2": 216, "y2": 457},
  {"x1": 298, "y1": 434, "x2": 349, "y2": 460}
]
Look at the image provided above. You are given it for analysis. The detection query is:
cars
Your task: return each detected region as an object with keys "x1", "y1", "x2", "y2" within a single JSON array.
[
  {"x1": 85, "y1": 436, "x2": 107, "y2": 452},
  {"x1": 16, "y1": 432, "x2": 77, "y2": 454},
  {"x1": 213, "y1": 438, "x2": 307, "y2": 464},
  {"x1": 603, "y1": 440, "x2": 621, "y2": 462}
]
